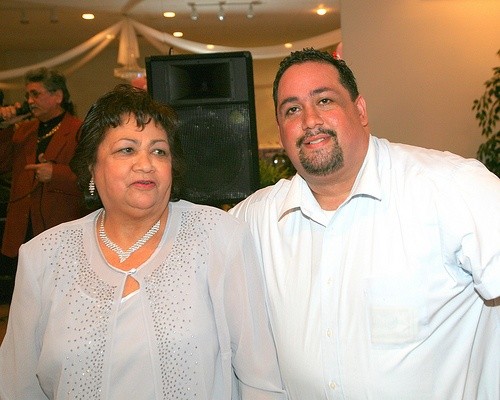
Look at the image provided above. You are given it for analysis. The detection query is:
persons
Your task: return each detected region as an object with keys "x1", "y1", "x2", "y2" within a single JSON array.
[
  {"x1": 0, "y1": 66, "x2": 91, "y2": 264},
  {"x1": 204, "y1": 45, "x2": 500, "y2": 400},
  {"x1": 0, "y1": 83, "x2": 227, "y2": 400}
]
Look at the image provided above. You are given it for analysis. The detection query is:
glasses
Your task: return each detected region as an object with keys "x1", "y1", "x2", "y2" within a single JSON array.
[{"x1": 24, "y1": 89, "x2": 50, "y2": 100}]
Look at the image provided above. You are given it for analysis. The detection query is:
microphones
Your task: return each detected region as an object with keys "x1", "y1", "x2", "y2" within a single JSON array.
[{"x1": 0, "y1": 105, "x2": 31, "y2": 123}]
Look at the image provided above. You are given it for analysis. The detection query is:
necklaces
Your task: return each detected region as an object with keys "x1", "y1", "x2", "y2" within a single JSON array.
[
  {"x1": 36, "y1": 119, "x2": 62, "y2": 142},
  {"x1": 98, "y1": 208, "x2": 164, "y2": 266}
]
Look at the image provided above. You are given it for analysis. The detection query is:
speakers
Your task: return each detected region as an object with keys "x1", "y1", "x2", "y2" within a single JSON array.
[{"x1": 144, "y1": 51, "x2": 260, "y2": 206}]
